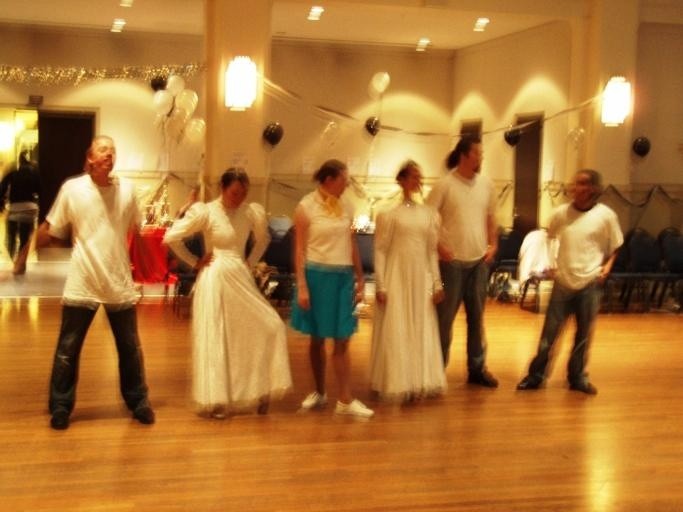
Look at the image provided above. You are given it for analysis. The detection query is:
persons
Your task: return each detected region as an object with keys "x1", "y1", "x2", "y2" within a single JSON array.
[
  {"x1": 160, "y1": 165, "x2": 295, "y2": 419},
  {"x1": 173, "y1": 183, "x2": 212, "y2": 221},
  {"x1": 9, "y1": 133, "x2": 156, "y2": 430},
  {"x1": 294, "y1": 158, "x2": 381, "y2": 420},
  {"x1": 510, "y1": 168, "x2": 625, "y2": 395},
  {"x1": 2, "y1": 150, "x2": 42, "y2": 274},
  {"x1": 427, "y1": 133, "x2": 500, "y2": 389},
  {"x1": 373, "y1": 162, "x2": 451, "y2": 405},
  {"x1": 515, "y1": 225, "x2": 559, "y2": 305}
]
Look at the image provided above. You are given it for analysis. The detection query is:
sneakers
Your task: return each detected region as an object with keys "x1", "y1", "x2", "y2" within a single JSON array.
[
  {"x1": 51, "y1": 412, "x2": 68, "y2": 429},
  {"x1": 469, "y1": 375, "x2": 499, "y2": 387},
  {"x1": 573, "y1": 382, "x2": 598, "y2": 395},
  {"x1": 135, "y1": 407, "x2": 155, "y2": 423},
  {"x1": 301, "y1": 391, "x2": 327, "y2": 409},
  {"x1": 517, "y1": 377, "x2": 536, "y2": 390},
  {"x1": 335, "y1": 399, "x2": 374, "y2": 418}
]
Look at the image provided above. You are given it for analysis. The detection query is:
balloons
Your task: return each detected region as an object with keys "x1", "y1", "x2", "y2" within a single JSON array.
[
  {"x1": 634, "y1": 136, "x2": 653, "y2": 159},
  {"x1": 148, "y1": 71, "x2": 207, "y2": 145},
  {"x1": 262, "y1": 122, "x2": 284, "y2": 144},
  {"x1": 503, "y1": 126, "x2": 524, "y2": 148},
  {"x1": 365, "y1": 116, "x2": 381, "y2": 138},
  {"x1": 371, "y1": 72, "x2": 390, "y2": 96}
]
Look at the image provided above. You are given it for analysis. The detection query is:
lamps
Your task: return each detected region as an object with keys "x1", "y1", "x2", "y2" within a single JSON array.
[
  {"x1": 601, "y1": 74, "x2": 632, "y2": 127},
  {"x1": 223, "y1": 56, "x2": 257, "y2": 112}
]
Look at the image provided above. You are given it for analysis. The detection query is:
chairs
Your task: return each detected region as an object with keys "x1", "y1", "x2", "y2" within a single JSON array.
[
  {"x1": 163, "y1": 214, "x2": 375, "y2": 318},
  {"x1": 486, "y1": 225, "x2": 683, "y2": 313}
]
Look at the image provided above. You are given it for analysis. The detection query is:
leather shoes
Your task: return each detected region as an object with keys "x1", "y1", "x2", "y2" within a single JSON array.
[
  {"x1": 259, "y1": 402, "x2": 268, "y2": 416},
  {"x1": 212, "y1": 413, "x2": 224, "y2": 419}
]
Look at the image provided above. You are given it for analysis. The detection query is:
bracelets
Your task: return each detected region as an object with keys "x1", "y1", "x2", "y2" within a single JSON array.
[{"x1": 355, "y1": 291, "x2": 366, "y2": 297}]
